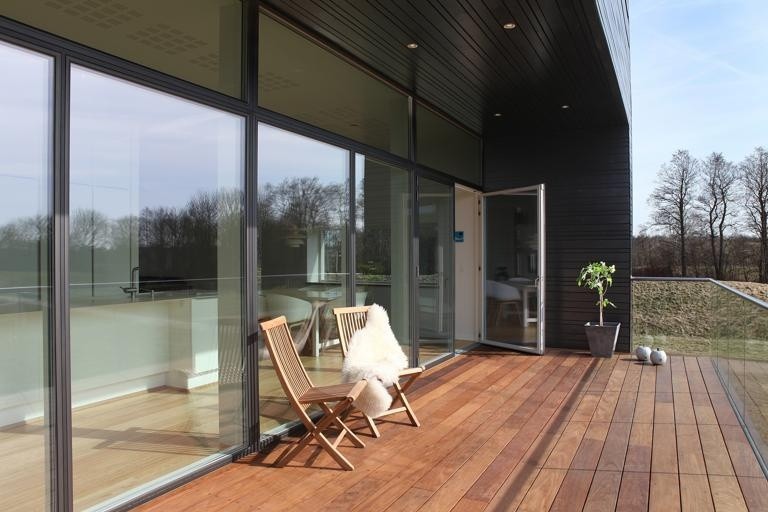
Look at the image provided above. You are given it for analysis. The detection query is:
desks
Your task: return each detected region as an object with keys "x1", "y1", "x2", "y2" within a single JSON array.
[
  {"x1": 504, "y1": 279, "x2": 539, "y2": 329},
  {"x1": 266, "y1": 285, "x2": 338, "y2": 356}
]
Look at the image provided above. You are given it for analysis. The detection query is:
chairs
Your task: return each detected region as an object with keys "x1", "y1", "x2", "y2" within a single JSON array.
[
  {"x1": 263, "y1": 292, "x2": 312, "y2": 357},
  {"x1": 485, "y1": 274, "x2": 522, "y2": 329},
  {"x1": 330, "y1": 304, "x2": 423, "y2": 439},
  {"x1": 258, "y1": 313, "x2": 367, "y2": 471}
]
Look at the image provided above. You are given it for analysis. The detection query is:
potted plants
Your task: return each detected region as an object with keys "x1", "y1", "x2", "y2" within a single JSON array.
[{"x1": 572, "y1": 260, "x2": 624, "y2": 360}]
